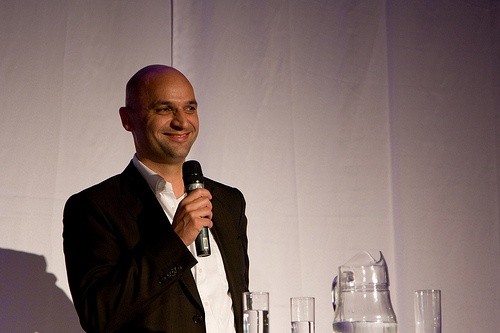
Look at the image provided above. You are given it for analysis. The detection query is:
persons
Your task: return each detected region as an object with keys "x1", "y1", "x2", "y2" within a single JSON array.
[{"x1": 62, "y1": 64, "x2": 253, "y2": 333}]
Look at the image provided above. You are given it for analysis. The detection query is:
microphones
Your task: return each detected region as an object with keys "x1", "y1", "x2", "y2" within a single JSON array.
[{"x1": 182, "y1": 160, "x2": 211, "y2": 257}]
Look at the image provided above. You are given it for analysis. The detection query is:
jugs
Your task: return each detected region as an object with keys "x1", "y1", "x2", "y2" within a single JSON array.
[{"x1": 331, "y1": 248, "x2": 398, "y2": 333}]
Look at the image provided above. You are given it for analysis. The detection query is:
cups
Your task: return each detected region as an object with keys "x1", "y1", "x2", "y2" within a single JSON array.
[
  {"x1": 242, "y1": 292, "x2": 269, "y2": 333},
  {"x1": 413, "y1": 290, "x2": 441, "y2": 333},
  {"x1": 290, "y1": 297, "x2": 315, "y2": 333}
]
